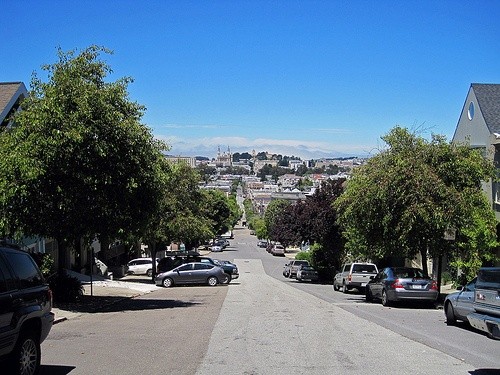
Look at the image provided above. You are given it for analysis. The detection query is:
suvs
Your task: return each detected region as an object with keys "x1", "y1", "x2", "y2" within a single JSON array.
[
  {"x1": 283, "y1": 260, "x2": 310, "y2": 278},
  {"x1": 0, "y1": 240, "x2": 56, "y2": 375},
  {"x1": 333, "y1": 262, "x2": 380, "y2": 294}
]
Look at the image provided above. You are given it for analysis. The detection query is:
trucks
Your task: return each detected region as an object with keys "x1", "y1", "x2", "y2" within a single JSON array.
[{"x1": 443, "y1": 266, "x2": 500, "y2": 341}]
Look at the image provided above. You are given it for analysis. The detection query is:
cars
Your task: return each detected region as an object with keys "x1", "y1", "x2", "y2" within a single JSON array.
[
  {"x1": 367, "y1": 266, "x2": 440, "y2": 309},
  {"x1": 125, "y1": 257, "x2": 160, "y2": 277},
  {"x1": 154, "y1": 261, "x2": 226, "y2": 288},
  {"x1": 256, "y1": 238, "x2": 300, "y2": 256},
  {"x1": 296, "y1": 264, "x2": 320, "y2": 282},
  {"x1": 140, "y1": 229, "x2": 239, "y2": 285},
  {"x1": 249, "y1": 230, "x2": 256, "y2": 235}
]
